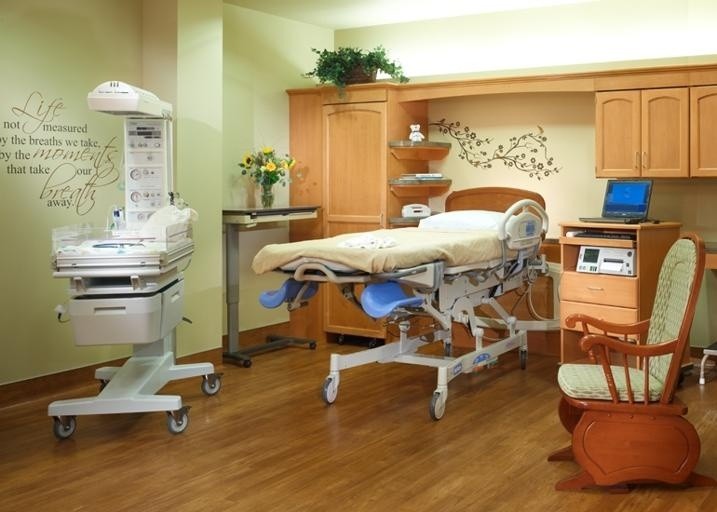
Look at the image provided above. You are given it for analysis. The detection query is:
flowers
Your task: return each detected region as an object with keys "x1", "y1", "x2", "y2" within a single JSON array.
[{"x1": 239, "y1": 146, "x2": 295, "y2": 188}]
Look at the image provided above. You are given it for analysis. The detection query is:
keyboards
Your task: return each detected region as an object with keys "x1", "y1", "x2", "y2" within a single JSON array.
[{"x1": 574, "y1": 232, "x2": 636, "y2": 240}]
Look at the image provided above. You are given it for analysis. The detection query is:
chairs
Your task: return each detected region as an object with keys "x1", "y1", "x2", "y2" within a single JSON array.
[{"x1": 547, "y1": 231, "x2": 709, "y2": 494}]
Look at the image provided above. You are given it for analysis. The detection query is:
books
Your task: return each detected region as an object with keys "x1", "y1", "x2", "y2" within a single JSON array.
[{"x1": 398, "y1": 173, "x2": 444, "y2": 180}]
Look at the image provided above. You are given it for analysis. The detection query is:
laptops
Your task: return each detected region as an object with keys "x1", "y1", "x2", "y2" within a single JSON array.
[{"x1": 579, "y1": 179, "x2": 654, "y2": 222}]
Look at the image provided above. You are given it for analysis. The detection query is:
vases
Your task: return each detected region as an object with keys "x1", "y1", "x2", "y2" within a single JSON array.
[{"x1": 260, "y1": 185, "x2": 276, "y2": 210}]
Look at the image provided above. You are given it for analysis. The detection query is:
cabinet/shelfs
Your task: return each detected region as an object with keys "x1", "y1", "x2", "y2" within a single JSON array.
[
  {"x1": 285, "y1": 81, "x2": 429, "y2": 349},
  {"x1": 389, "y1": 140, "x2": 451, "y2": 226},
  {"x1": 595, "y1": 65, "x2": 690, "y2": 178},
  {"x1": 556, "y1": 220, "x2": 692, "y2": 390},
  {"x1": 690, "y1": 63, "x2": 717, "y2": 178}
]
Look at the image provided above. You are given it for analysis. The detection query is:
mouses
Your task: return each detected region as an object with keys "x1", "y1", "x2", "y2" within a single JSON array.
[{"x1": 653, "y1": 219, "x2": 660, "y2": 224}]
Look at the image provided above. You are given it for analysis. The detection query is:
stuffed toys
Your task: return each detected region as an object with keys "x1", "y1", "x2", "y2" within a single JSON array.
[{"x1": 408, "y1": 124, "x2": 425, "y2": 141}]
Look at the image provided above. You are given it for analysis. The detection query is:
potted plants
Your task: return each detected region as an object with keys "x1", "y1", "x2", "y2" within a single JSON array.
[{"x1": 304, "y1": 45, "x2": 410, "y2": 98}]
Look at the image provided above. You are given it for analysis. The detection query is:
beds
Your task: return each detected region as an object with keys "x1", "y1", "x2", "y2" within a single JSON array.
[{"x1": 250, "y1": 187, "x2": 549, "y2": 421}]
